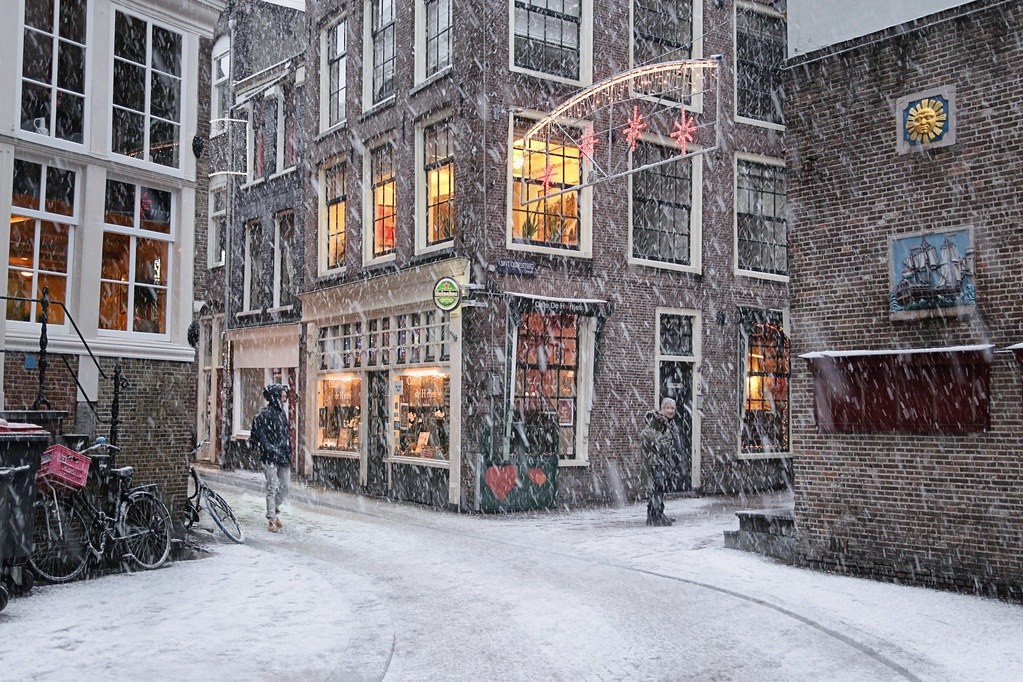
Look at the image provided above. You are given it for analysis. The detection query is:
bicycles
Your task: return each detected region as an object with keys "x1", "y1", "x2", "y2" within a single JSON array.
[
  {"x1": 23, "y1": 438, "x2": 175, "y2": 582},
  {"x1": 185, "y1": 436, "x2": 246, "y2": 545}
]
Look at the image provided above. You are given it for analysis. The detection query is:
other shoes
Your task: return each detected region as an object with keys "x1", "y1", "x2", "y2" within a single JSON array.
[
  {"x1": 276, "y1": 518, "x2": 282, "y2": 528},
  {"x1": 268, "y1": 518, "x2": 277, "y2": 532},
  {"x1": 647, "y1": 514, "x2": 676, "y2": 526}
]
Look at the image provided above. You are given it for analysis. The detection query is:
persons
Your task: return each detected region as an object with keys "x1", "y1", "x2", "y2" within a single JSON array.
[
  {"x1": 249, "y1": 381, "x2": 293, "y2": 533},
  {"x1": 639, "y1": 398, "x2": 683, "y2": 527}
]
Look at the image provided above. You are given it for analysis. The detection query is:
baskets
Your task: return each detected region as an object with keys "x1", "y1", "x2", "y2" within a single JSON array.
[
  {"x1": 34, "y1": 444, "x2": 91, "y2": 494},
  {"x1": 85, "y1": 453, "x2": 116, "y2": 485}
]
